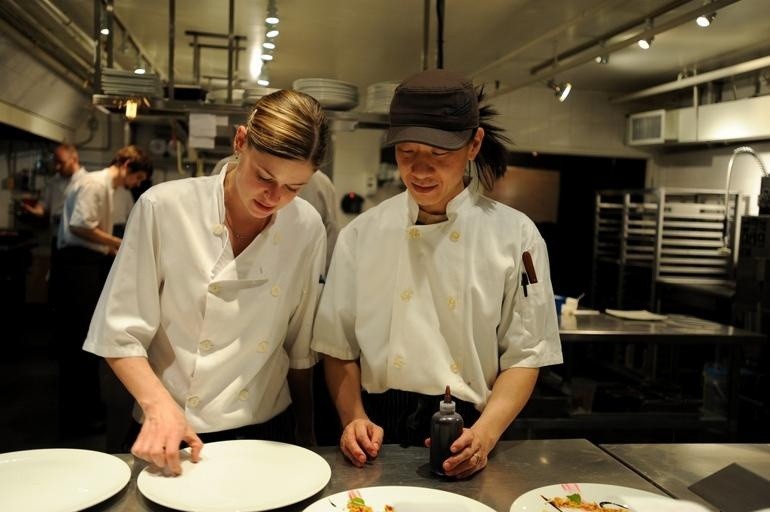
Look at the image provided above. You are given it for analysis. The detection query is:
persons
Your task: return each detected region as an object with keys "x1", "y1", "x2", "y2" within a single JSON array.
[
  {"x1": 310, "y1": 70, "x2": 565, "y2": 479},
  {"x1": 82, "y1": 88, "x2": 327, "y2": 476},
  {"x1": 22, "y1": 143, "x2": 153, "y2": 443},
  {"x1": 210, "y1": 155, "x2": 341, "y2": 278}
]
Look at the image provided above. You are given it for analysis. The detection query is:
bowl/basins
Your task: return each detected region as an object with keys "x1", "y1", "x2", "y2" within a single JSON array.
[{"x1": 243, "y1": 86, "x2": 280, "y2": 104}]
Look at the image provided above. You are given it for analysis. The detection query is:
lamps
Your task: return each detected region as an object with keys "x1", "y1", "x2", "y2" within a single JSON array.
[
  {"x1": 99, "y1": 2, "x2": 157, "y2": 80},
  {"x1": 256, "y1": 0, "x2": 281, "y2": 89},
  {"x1": 539, "y1": 0, "x2": 719, "y2": 108}
]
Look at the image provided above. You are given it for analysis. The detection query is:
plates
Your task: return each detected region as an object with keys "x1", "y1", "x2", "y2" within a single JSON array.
[
  {"x1": 606, "y1": 307, "x2": 669, "y2": 322},
  {"x1": 294, "y1": 77, "x2": 403, "y2": 112},
  {"x1": 2, "y1": 448, "x2": 130, "y2": 509},
  {"x1": 509, "y1": 482, "x2": 709, "y2": 512},
  {"x1": 100, "y1": 66, "x2": 161, "y2": 99},
  {"x1": 138, "y1": 437, "x2": 331, "y2": 511},
  {"x1": 299, "y1": 486, "x2": 493, "y2": 512}
]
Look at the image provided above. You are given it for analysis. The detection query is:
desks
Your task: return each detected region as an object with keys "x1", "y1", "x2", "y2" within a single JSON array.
[
  {"x1": 597, "y1": 439, "x2": 768, "y2": 512},
  {"x1": 506, "y1": 309, "x2": 757, "y2": 440},
  {"x1": 86, "y1": 427, "x2": 675, "y2": 512}
]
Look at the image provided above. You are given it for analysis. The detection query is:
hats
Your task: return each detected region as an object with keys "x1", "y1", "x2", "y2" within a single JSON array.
[{"x1": 384, "y1": 68, "x2": 480, "y2": 150}]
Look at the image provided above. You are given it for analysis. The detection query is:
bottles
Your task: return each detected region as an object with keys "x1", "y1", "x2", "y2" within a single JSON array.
[{"x1": 428, "y1": 384, "x2": 465, "y2": 477}]
